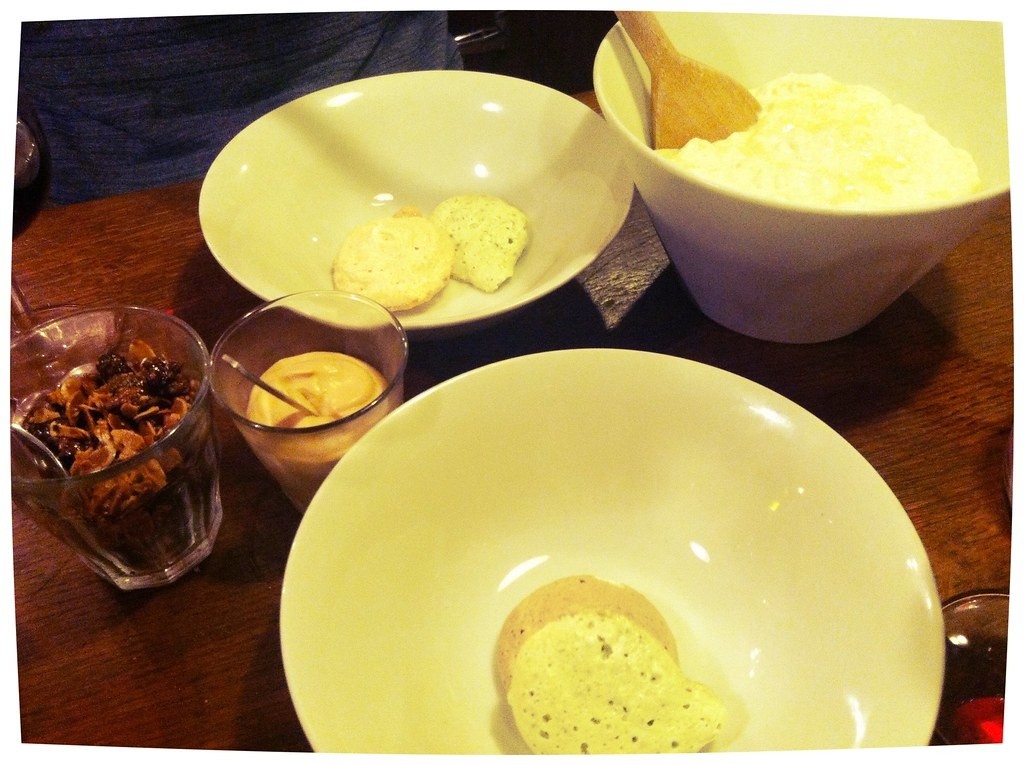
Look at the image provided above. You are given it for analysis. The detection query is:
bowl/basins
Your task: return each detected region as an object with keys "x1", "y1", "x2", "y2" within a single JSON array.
[
  {"x1": 280, "y1": 347, "x2": 946, "y2": 754},
  {"x1": 197, "y1": 71, "x2": 635, "y2": 341}
]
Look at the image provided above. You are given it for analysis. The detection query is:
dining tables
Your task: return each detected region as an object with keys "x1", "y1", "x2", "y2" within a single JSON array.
[{"x1": 8, "y1": 95, "x2": 1017, "y2": 753}]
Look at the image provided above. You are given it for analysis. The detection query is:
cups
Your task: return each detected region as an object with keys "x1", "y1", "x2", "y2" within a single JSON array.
[
  {"x1": 593, "y1": 11, "x2": 1009, "y2": 343},
  {"x1": 934, "y1": 589, "x2": 1009, "y2": 746},
  {"x1": 11, "y1": 305, "x2": 222, "y2": 592},
  {"x1": 207, "y1": 289, "x2": 408, "y2": 515}
]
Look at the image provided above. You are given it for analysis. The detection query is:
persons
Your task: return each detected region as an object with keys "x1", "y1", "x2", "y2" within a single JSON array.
[{"x1": 13, "y1": 9, "x2": 467, "y2": 210}]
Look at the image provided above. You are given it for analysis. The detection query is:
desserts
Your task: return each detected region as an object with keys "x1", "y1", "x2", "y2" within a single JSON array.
[
  {"x1": 428, "y1": 193, "x2": 530, "y2": 293},
  {"x1": 331, "y1": 205, "x2": 455, "y2": 312},
  {"x1": 243, "y1": 351, "x2": 398, "y2": 512},
  {"x1": 650, "y1": 67, "x2": 982, "y2": 215},
  {"x1": 494, "y1": 574, "x2": 729, "y2": 755}
]
